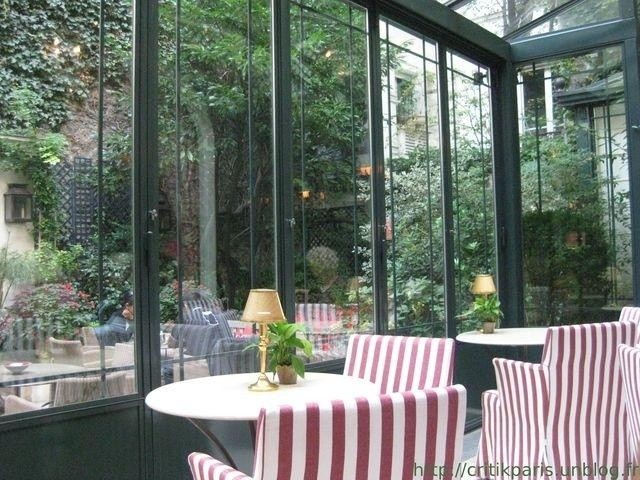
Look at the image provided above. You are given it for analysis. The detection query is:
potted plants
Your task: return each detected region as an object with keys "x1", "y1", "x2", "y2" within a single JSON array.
[{"x1": 471, "y1": 293, "x2": 505, "y2": 334}]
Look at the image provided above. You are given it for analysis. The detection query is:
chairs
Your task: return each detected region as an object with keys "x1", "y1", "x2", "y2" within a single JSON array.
[{"x1": 1, "y1": 299, "x2": 640, "y2": 480}]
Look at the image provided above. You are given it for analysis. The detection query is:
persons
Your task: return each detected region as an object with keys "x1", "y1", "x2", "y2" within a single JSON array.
[
  {"x1": 110, "y1": 295, "x2": 134, "y2": 346},
  {"x1": 305, "y1": 246, "x2": 351, "y2": 303}
]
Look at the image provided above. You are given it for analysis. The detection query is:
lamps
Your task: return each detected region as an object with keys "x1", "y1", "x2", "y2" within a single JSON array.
[
  {"x1": 239, "y1": 287, "x2": 287, "y2": 392},
  {"x1": 470, "y1": 275, "x2": 497, "y2": 299}
]
